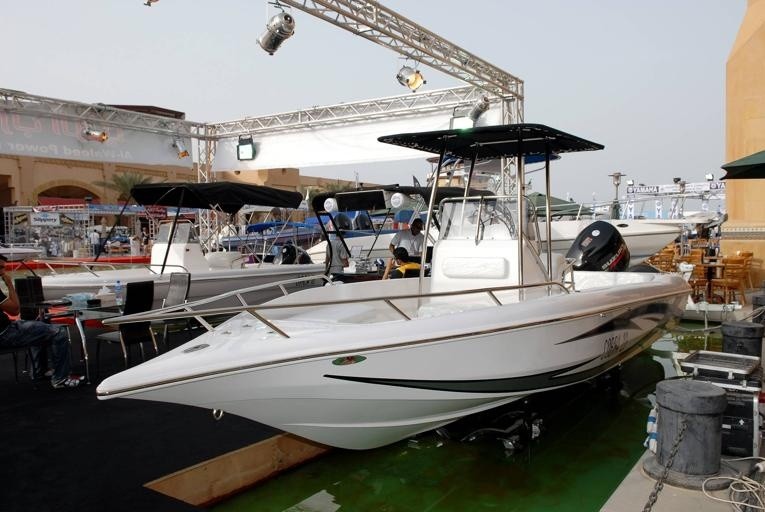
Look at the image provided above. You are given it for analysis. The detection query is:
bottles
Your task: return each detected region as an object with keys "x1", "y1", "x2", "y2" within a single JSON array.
[{"x1": 115, "y1": 281, "x2": 122, "y2": 300}]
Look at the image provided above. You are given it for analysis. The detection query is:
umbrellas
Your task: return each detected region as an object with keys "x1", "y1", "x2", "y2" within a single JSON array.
[{"x1": 719, "y1": 150, "x2": 764, "y2": 180}]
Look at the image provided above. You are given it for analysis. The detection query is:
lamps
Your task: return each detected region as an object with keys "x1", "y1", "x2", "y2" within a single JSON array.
[
  {"x1": 258, "y1": 2, "x2": 428, "y2": 97},
  {"x1": 81, "y1": 121, "x2": 255, "y2": 162}
]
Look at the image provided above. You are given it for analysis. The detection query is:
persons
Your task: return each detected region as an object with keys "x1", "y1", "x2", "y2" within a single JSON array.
[
  {"x1": 381, "y1": 247, "x2": 421, "y2": 281},
  {"x1": 0, "y1": 254, "x2": 88, "y2": 389},
  {"x1": 388, "y1": 218, "x2": 424, "y2": 264},
  {"x1": 325, "y1": 227, "x2": 349, "y2": 276},
  {"x1": 141, "y1": 226, "x2": 150, "y2": 255},
  {"x1": 90, "y1": 230, "x2": 100, "y2": 254}
]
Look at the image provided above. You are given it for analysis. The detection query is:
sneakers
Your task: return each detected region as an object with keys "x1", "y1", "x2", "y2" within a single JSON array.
[
  {"x1": 31, "y1": 368, "x2": 55, "y2": 379},
  {"x1": 50, "y1": 373, "x2": 88, "y2": 388}
]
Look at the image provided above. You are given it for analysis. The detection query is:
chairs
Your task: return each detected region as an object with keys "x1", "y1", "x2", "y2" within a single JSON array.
[
  {"x1": 648, "y1": 239, "x2": 754, "y2": 306},
  {"x1": 0, "y1": 272, "x2": 191, "y2": 387}
]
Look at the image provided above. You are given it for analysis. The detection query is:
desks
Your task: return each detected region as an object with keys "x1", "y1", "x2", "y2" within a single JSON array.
[{"x1": 331, "y1": 273, "x2": 382, "y2": 284}]
